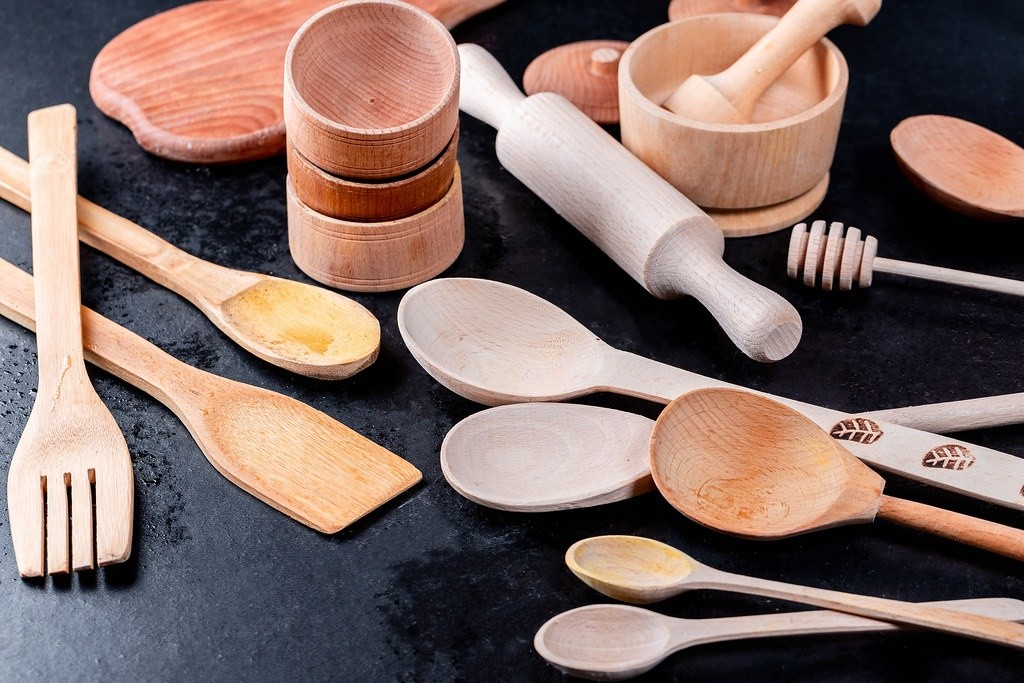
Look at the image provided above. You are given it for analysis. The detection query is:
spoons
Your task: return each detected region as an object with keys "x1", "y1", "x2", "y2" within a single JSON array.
[
  {"x1": 648, "y1": 386, "x2": 1024, "y2": 561},
  {"x1": 439, "y1": 392, "x2": 1023, "y2": 513},
  {"x1": 564, "y1": 534, "x2": 1024, "y2": 649},
  {"x1": 532, "y1": 597, "x2": 1024, "y2": 680},
  {"x1": 889, "y1": 113, "x2": 1024, "y2": 223},
  {"x1": 1, "y1": 145, "x2": 381, "y2": 382},
  {"x1": 396, "y1": 274, "x2": 1023, "y2": 515}
]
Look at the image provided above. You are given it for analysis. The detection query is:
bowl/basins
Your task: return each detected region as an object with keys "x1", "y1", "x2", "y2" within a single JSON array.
[
  {"x1": 282, "y1": 0, "x2": 465, "y2": 292},
  {"x1": 618, "y1": 11, "x2": 849, "y2": 211}
]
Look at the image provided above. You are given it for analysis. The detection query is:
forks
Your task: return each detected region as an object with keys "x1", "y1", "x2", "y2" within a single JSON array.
[{"x1": 7, "y1": 101, "x2": 135, "y2": 583}]
